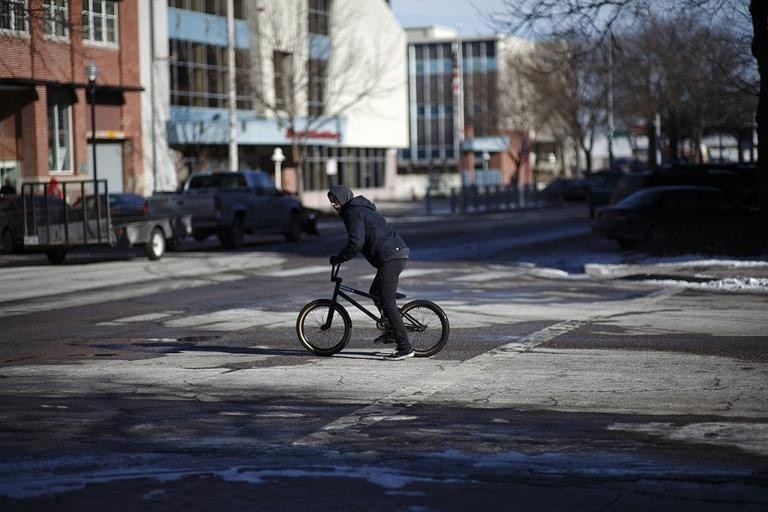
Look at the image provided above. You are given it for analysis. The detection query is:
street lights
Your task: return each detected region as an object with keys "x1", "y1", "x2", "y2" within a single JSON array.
[{"x1": 86, "y1": 61, "x2": 100, "y2": 195}]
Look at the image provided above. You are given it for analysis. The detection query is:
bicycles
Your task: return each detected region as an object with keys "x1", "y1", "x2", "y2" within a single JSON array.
[{"x1": 295, "y1": 258, "x2": 450, "y2": 359}]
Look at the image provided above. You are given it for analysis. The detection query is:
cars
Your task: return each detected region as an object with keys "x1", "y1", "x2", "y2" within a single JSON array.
[{"x1": 586, "y1": 158, "x2": 767, "y2": 254}]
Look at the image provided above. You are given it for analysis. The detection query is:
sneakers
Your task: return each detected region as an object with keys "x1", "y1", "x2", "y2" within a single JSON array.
[
  {"x1": 384, "y1": 349, "x2": 414, "y2": 360},
  {"x1": 373, "y1": 333, "x2": 394, "y2": 345}
]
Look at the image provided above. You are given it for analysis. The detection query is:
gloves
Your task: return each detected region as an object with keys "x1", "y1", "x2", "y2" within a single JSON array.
[{"x1": 330, "y1": 255, "x2": 340, "y2": 265}]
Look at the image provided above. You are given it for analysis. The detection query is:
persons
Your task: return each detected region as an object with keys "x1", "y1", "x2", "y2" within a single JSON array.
[
  {"x1": 46, "y1": 177, "x2": 62, "y2": 201},
  {"x1": 1, "y1": 177, "x2": 16, "y2": 196},
  {"x1": 327, "y1": 185, "x2": 415, "y2": 361}
]
